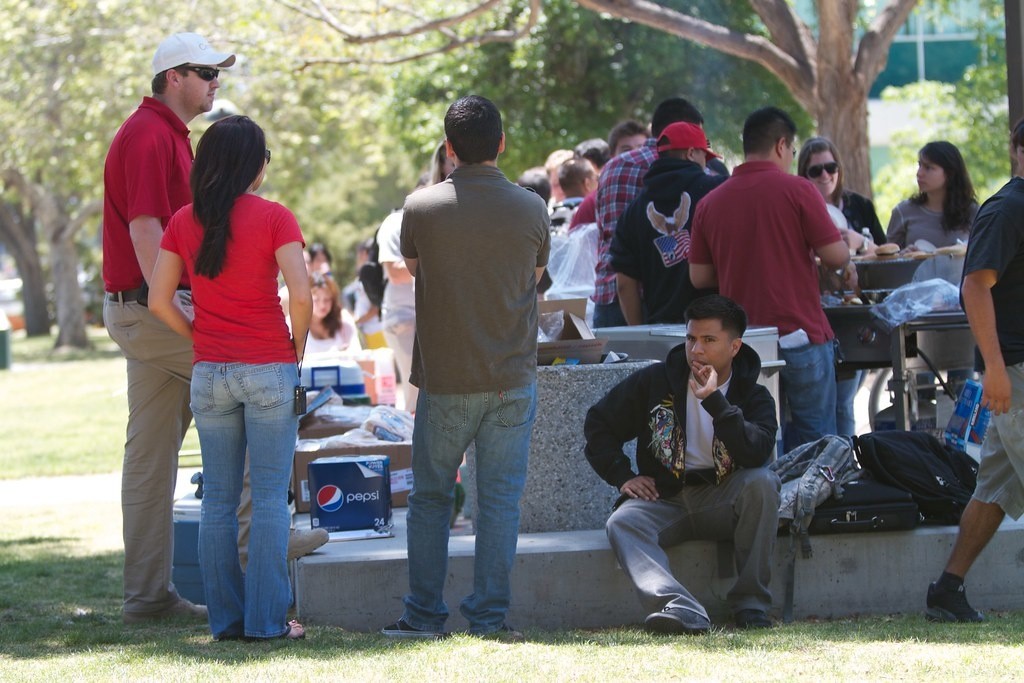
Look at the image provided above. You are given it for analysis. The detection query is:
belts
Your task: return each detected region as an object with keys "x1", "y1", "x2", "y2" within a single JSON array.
[{"x1": 109, "y1": 288, "x2": 139, "y2": 304}]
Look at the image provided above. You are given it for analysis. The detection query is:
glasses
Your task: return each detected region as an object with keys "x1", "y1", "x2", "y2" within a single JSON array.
[
  {"x1": 263, "y1": 148, "x2": 271, "y2": 164},
  {"x1": 808, "y1": 162, "x2": 839, "y2": 178},
  {"x1": 184, "y1": 66, "x2": 220, "y2": 81}
]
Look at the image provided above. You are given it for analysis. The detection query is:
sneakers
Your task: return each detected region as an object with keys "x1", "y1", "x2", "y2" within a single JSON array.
[
  {"x1": 645, "y1": 608, "x2": 713, "y2": 634},
  {"x1": 925, "y1": 579, "x2": 986, "y2": 624},
  {"x1": 124, "y1": 601, "x2": 210, "y2": 623},
  {"x1": 237, "y1": 526, "x2": 329, "y2": 571},
  {"x1": 737, "y1": 609, "x2": 773, "y2": 629},
  {"x1": 381, "y1": 618, "x2": 443, "y2": 636},
  {"x1": 471, "y1": 625, "x2": 522, "y2": 639}
]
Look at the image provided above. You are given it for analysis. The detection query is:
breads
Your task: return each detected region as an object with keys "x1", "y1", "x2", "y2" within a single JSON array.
[{"x1": 301, "y1": 390, "x2": 415, "y2": 449}]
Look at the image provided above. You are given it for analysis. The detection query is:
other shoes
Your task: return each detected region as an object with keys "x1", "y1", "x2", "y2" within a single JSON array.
[{"x1": 218, "y1": 623, "x2": 306, "y2": 641}]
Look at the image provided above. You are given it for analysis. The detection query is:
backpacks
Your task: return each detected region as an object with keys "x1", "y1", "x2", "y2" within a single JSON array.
[
  {"x1": 767, "y1": 433, "x2": 865, "y2": 561},
  {"x1": 854, "y1": 430, "x2": 980, "y2": 524}
]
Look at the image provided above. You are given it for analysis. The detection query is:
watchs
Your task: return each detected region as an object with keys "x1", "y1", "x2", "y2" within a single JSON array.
[{"x1": 859, "y1": 236, "x2": 870, "y2": 254}]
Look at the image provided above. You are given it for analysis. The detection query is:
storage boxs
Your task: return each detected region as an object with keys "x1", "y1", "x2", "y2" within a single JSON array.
[
  {"x1": 172, "y1": 493, "x2": 206, "y2": 605},
  {"x1": 537, "y1": 297, "x2": 609, "y2": 364},
  {"x1": 292, "y1": 360, "x2": 413, "y2": 542}
]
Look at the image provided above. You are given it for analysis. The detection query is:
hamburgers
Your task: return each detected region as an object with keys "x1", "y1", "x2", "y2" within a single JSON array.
[{"x1": 875, "y1": 243, "x2": 901, "y2": 260}]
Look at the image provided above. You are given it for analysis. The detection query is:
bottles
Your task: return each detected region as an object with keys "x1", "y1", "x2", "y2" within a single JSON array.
[{"x1": 859, "y1": 227, "x2": 874, "y2": 255}]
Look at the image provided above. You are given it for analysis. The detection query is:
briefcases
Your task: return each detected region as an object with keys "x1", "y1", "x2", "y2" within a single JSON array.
[{"x1": 811, "y1": 479, "x2": 921, "y2": 534}]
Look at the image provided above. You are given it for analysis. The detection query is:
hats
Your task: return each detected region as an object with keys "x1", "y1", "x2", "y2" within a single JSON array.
[
  {"x1": 657, "y1": 121, "x2": 723, "y2": 162},
  {"x1": 153, "y1": 32, "x2": 236, "y2": 75}
]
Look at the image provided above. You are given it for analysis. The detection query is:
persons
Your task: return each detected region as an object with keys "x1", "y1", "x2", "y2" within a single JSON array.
[
  {"x1": 885, "y1": 140, "x2": 981, "y2": 400},
  {"x1": 381, "y1": 96, "x2": 551, "y2": 641},
  {"x1": 688, "y1": 107, "x2": 850, "y2": 455},
  {"x1": 148, "y1": 116, "x2": 313, "y2": 643},
  {"x1": 103, "y1": 33, "x2": 328, "y2": 626},
  {"x1": 517, "y1": 99, "x2": 730, "y2": 342},
  {"x1": 798, "y1": 137, "x2": 887, "y2": 465},
  {"x1": 277, "y1": 138, "x2": 466, "y2": 423},
  {"x1": 584, "y1": 293, "x2": 781, "y2": 635},
  {"x1": 924, "y1": 117, "x2": 1024, "y2": 623}
]
